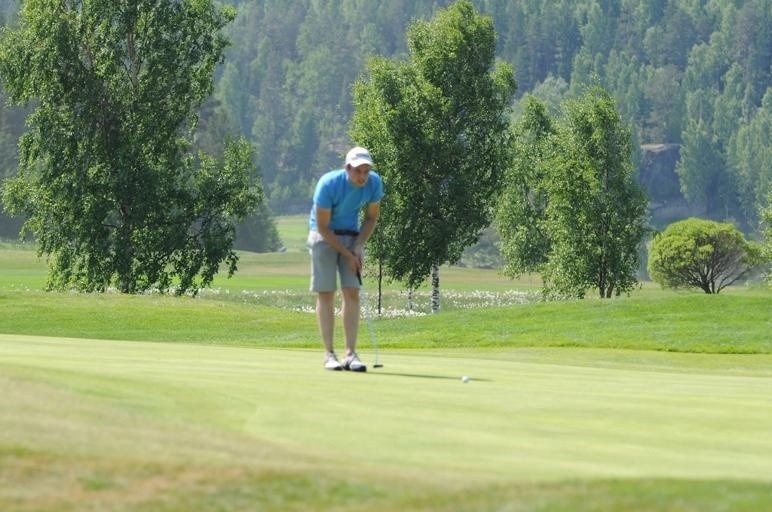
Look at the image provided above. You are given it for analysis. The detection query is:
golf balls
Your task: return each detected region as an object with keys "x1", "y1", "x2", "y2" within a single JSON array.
[{"x1": 462, "y1": 377, "x2": 469, "y2": 383}]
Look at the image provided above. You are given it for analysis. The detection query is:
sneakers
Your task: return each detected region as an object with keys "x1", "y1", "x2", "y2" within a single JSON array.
[
  {"x1": 324, "y1": 351, "x2": 344, "y2": 370},
  {"x1": 343, "y1": 352, "x2": 367, "y2": 371}
]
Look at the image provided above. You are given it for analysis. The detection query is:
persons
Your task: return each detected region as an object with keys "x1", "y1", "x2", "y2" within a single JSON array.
[{"x1": 305, "y1": 146, "x2": 386, "y2": 373}]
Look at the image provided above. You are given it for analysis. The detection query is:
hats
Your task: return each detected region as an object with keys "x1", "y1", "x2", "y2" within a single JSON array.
[{"x1": 345, "y1": 146, "x2": 374, "y2": 169}]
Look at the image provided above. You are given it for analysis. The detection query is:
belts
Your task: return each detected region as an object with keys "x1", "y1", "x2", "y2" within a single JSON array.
[{"x1": 334, "y1": 228, "x2": 360, "y2": 236}]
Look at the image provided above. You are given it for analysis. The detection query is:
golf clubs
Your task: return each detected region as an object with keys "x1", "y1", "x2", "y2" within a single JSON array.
[{"x1": 357, "y1": 271, "x2": 383, "y2": 368}]
